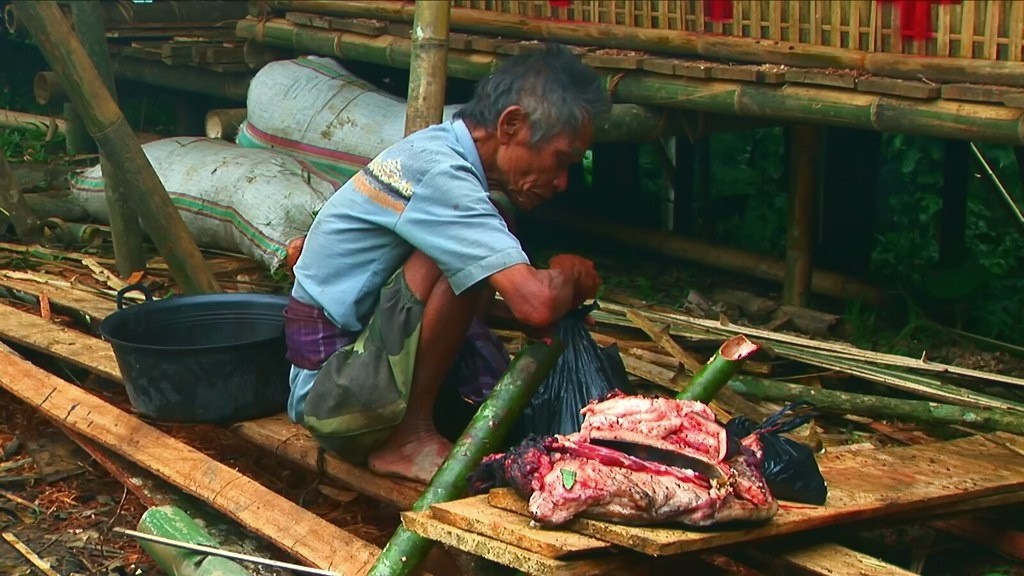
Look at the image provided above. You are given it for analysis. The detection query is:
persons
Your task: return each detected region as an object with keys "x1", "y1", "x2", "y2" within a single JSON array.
[{"x1": 283, "y1": 44, "x2": 613, "y2": 485}]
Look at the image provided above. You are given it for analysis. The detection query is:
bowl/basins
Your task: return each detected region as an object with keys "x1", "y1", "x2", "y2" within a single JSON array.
[{"x1": 96, "y1": 284, "x2": 291, "y2": 423}]
